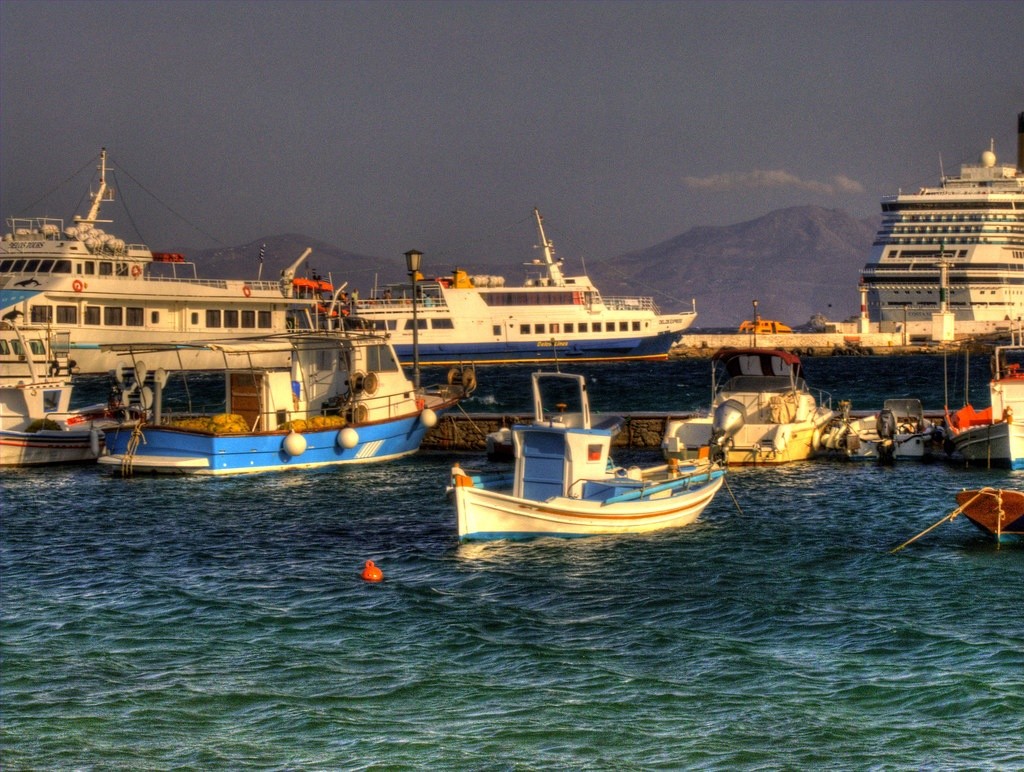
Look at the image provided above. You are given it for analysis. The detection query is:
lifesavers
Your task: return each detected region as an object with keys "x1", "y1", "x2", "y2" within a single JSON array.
[
  {"x1": 72, "y1": 279, "x2": 83, "y2": 291},
  {"x1": 776, "y1": 345, "x2": 877, "y2": 357},
  {"x1": 243, "y1": 286, "x2": 251, "y2": 297},
  {"x1": 310, "y1": 303, "x2": 349, "y2": 319},
  {"x1": 131, "y1": 265, "x2": 141, "y2": 276}
]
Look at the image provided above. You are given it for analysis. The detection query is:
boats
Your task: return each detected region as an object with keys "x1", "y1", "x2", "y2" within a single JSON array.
[
  {"x1": 88, "y1": 282, "x2": 476, "y2": 479},
  {"x1": 0, "y1": 137, "x2": 1024, "y2": 487},
  {"x1": 444, "y1": 373, "x2": 731, "y2": 549},
  {"x1": 293, "y1": 277, "x2": 332, "y2": 292},
  {"x1": 310, "y1": 303, "x2": 350, "y2": 318},
  {"x1": 955, "y1": 486, "x2": 1024, "y2": 541}
]
